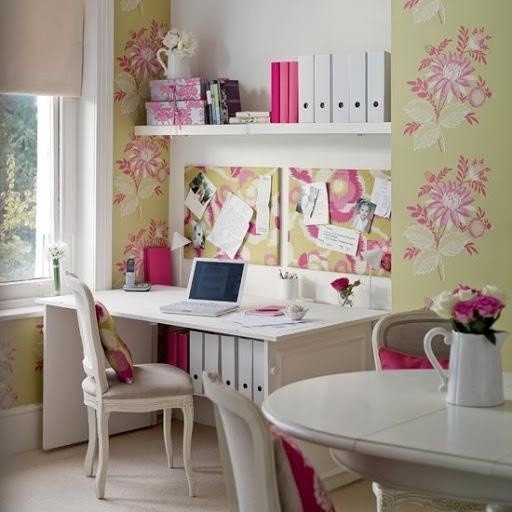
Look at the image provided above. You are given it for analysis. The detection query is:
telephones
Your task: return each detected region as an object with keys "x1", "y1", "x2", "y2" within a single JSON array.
[{"x1": 122, "y1": 257, "x2": 151, "y2": 291}]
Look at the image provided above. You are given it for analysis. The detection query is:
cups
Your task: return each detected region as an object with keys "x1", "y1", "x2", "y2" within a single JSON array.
[{"x1": 282, "y1": 279, "x2": 299, "y2": 300}]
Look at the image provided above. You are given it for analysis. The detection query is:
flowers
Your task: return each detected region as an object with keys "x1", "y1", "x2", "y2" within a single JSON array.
[
  {"x1": 112, "y1": 0, "x2": 171, "y2": 283},
  {"x1": 163, "y1": 29, "x2": 198, "y2": 58},
  {"x1": 401, "y1": 0, "x2": 492, "y2": 294},
  {"x1": 47, "y1": 241, "x2": 67, "y2": 260},
  {"x1": 430, "y1": 284, "x2": 506, "y2": 346},
  {"x1": 0, "y1": 324, "x2": 43, "y2": 410},
  {"x1": 331, "y1": 278, "x2": 361, "y2": 307}
]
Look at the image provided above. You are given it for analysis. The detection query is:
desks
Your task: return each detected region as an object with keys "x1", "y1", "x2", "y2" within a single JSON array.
[
  {"x1": 36, "y1": 285, "x2": 390, "y2": 493},
  {"x1": 262, "y1": 369, "x2": 512, "y2": 506}
]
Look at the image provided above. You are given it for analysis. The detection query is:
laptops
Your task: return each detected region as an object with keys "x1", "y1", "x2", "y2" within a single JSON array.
[{"x1": 161, "y1": 258, "x2": 248, "y2": 317}]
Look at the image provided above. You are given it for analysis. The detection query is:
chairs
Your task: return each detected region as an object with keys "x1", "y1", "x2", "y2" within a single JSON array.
[
  {"x1": 200, "y1": 370, "x2": 281, "y2": 512},
  {"x1": 65, "y1": 271, "x2": 195, "y2": 499},
  {"x1": 371, "y1": 312, "x2": 503, "y2": 512}
]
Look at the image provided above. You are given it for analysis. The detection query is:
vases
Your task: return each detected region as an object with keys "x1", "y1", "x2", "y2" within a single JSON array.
[
  {"x1": 157, "y1": 48, "x2": 193, "y2": 79},
  {"x1": 53, "y1": 260, "x2": 60, "y2": 295}
]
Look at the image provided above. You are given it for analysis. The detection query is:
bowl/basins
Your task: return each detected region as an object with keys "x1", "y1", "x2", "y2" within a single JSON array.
[{"x1": 281, "y1": 308, "x2": 309, "y2": 320}]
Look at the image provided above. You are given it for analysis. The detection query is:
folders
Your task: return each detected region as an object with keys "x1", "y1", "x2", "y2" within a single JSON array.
[
  {"x1": 186, "y1": 329, "x2": 266, "y2": 411},
  {"x1": 296, "y1": 50, "x2": 392, "y2": 122}
]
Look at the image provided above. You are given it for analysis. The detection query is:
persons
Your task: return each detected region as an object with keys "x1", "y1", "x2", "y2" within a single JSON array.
[
  {"x1": 192, "y1": 184, "x2": 209, "y2": 204},
  {"x1": 353, "y1": 198, "x2": 371, "y2": 231}
]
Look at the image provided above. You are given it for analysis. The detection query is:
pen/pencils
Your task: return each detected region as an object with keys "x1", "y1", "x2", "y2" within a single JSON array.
[{"x1": 278, "y1": 268, "x2": 298, "y2": 279}]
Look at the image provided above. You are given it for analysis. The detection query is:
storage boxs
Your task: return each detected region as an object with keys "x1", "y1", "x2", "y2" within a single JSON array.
[
  {"x1": 146, "y1": 100, "x2": 207, "y2": 125},
  {"x1": 147, "y1": 78, "x2": 208, "y2": 100}
]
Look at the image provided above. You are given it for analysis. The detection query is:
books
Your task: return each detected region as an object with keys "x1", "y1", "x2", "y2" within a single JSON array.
[{"x1": 206, "y1": 77, "x2": 270, "y2": 124}]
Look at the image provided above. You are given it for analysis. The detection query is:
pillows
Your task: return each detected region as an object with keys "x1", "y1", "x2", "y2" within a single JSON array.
[
  {"x1": 378, "y1": 346, "x2": 449, "y2": 370},
  {"x1": 95, "y1": 301, "x2": 135, "y2": 385},
  {"x1": 272, "y1": 431, "x2": 334, "y2": 512}
]
